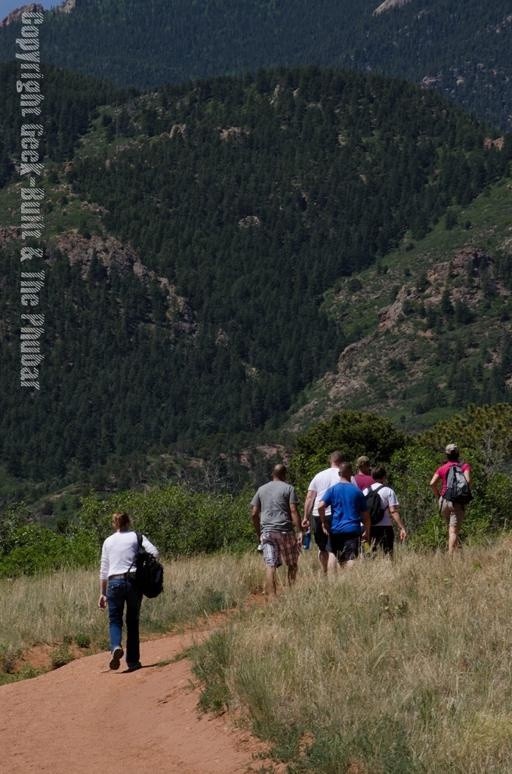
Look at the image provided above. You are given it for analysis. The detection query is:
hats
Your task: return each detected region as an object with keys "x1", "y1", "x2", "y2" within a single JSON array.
[{"x1": 445, "y1": 444, "x2": 459, "y2": 454}]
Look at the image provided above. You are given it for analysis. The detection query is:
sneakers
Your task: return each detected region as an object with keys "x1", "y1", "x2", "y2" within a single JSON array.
[
  {"x1": 128, "y1": 662, "x2": 141, "y2": 671},
  {"x1": 110, "y1": 646, "x2": 124, "y2": 670}
]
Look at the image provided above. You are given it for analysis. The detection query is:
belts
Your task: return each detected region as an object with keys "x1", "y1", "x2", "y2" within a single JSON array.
[{"x1": 109, "y1": 572, "x2": 136, "y2": 580}]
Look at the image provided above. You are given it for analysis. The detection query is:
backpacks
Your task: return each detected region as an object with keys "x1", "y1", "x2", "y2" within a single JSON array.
[
  {"x1": 442, "y1": 463, "x2": 474, "y2": 505},
  {"x1": 364, "y1": 485, "x2": 389, "y2": 525},
  {"x1": 125, "y1": 531, "x2": 163, "y2": 598}
]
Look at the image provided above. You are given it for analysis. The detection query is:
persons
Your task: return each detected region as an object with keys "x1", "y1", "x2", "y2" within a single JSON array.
[
  {"x1": 250, "y1": 463, "x2": 304, "y2": 596},
  {"x1": 317, "y1": 463, "x2": 368, "y2": 574},
  {"x1": 352, "y1": 456, "x2": 376, "y2": 491},
  {"x1": 97, "y1": 511, "x2": 160, "y2": 672},
  {"x1": 429, "y1": 443, "x2": 471, "y2": 556},
  {"x1": 362, "y1": 467, "x2": 407, "y2": 562},
  {"x1": 301, "y1": 450, "x2": 345, "y2": 576}
]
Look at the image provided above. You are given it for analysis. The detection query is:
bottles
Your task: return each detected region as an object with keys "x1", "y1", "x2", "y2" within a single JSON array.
[
  {"x1": 362, "y1": 539, "x2": 373, "y2": 561},
  {"x1": 301, "y1": 526, "x2": 312, "y2": 550}
]
